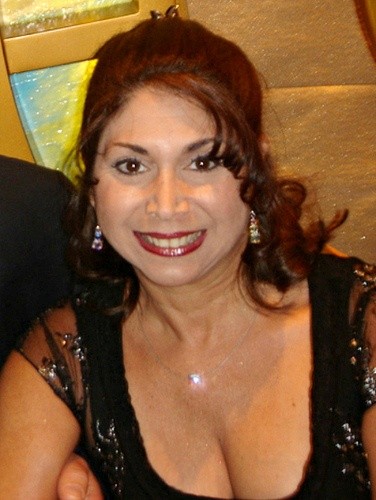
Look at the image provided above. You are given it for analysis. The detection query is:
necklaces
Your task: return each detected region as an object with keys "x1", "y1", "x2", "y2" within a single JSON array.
[{"x1": 135, "y1": 285, "x2": 264, "y2": 386}]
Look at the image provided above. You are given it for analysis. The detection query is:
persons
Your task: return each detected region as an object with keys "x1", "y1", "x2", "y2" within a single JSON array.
[
  {"x1": 0, "y1": 5, "x2": 376, "y2": 497},
  {"x1": 1, "y1": 149, "x2": 106, "y2": 499}
]
